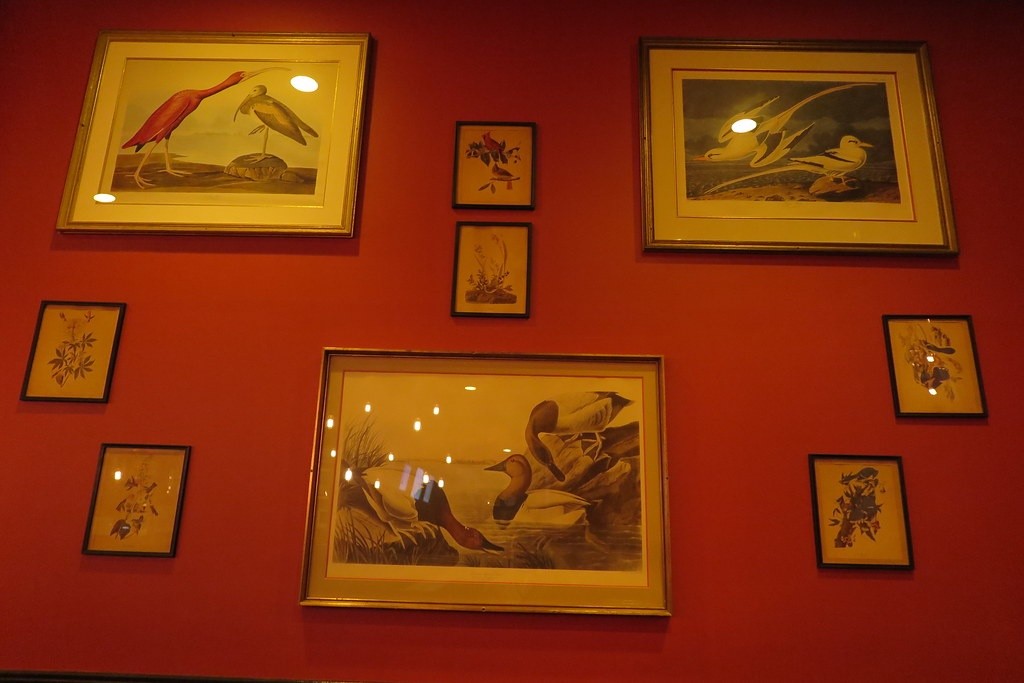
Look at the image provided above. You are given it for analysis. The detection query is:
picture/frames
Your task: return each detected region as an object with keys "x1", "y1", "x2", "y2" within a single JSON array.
[
  {"x1": 54, "y1": 27, "x2": 374, "y2": 240},
  {"x1": 450, "y1": 221, "x2": 533, "y2": 320},
  {"x1": 637, "y1": 34, "x2": 960, "y2": 259},
  {"x1": 808, "y1": 453, "x2": 917, "y2": 573},
  {"x1": 882, "y1": 312, "x2": 991, "y2": 420},
  {"x1": 451, "y1": 121, "x2": 537, "y2": 211},
  {"x1": 298, "y1": 346, "x2": 674, "y2": 618},
  {"x1": 19, "y1": 300, "x2": 127, "y2": 403},
  {"x1": 81, "y1": 442, "x2": 193, "y2": 559}
]
